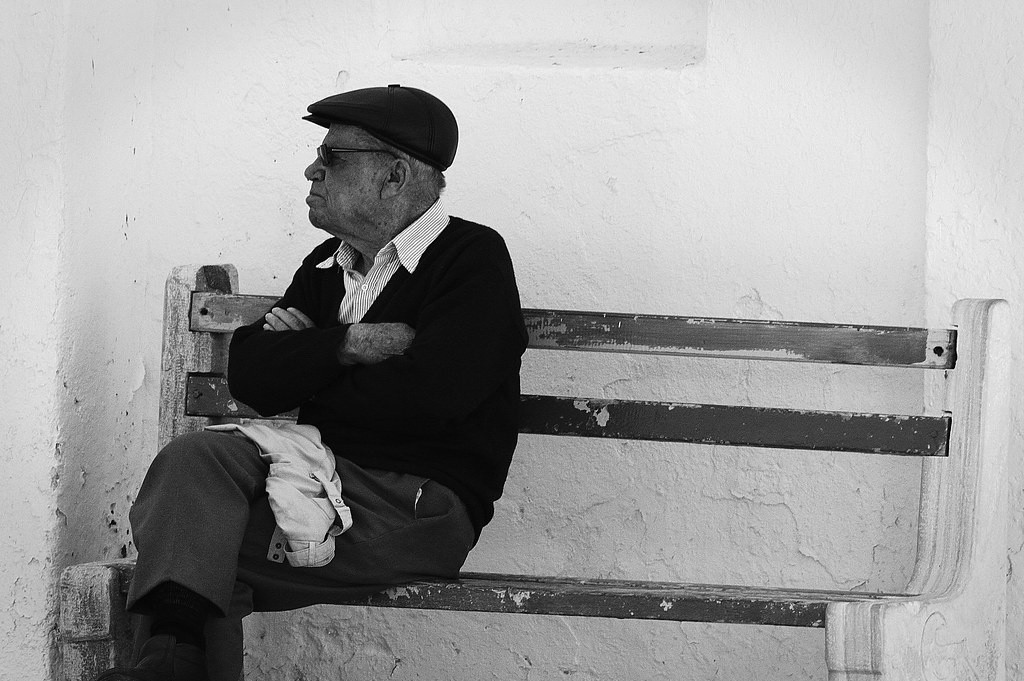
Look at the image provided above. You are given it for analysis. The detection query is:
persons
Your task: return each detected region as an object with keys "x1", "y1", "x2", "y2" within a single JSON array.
[{"x1": 83, "y1": 83, "x2": 530, "y2": 681}]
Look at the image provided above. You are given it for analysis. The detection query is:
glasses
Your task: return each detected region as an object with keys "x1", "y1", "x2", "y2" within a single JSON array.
[{"x1": 316, "y1": 143, "x2": 402, "y2": 168}]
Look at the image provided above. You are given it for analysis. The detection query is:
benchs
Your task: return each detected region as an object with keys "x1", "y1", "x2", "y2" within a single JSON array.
[{"x1": 64, "y1": 265, "x2": 1010, "y2": 681}]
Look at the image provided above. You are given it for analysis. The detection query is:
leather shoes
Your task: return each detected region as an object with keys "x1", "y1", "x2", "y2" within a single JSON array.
[{"x1": 89, "y1": 634, "x2": 211, "y2": 681}]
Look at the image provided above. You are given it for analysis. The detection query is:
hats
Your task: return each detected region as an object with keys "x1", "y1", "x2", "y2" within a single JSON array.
[{"x1": 301, "y1": 85, "x2": 459, "y2": 172}]
